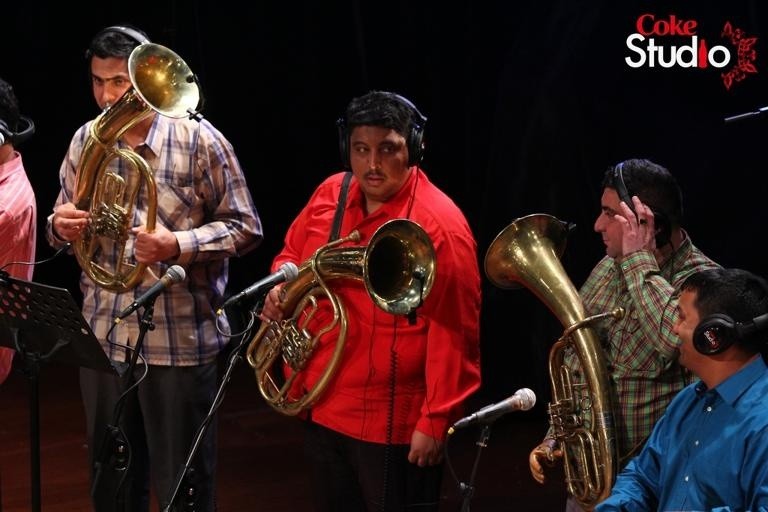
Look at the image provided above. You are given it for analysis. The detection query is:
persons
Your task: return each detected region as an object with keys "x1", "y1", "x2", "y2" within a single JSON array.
[
  {"x1": 528, "y1": 157, "x2": 728, "y2": 512},
  {"x1": 45, "y1": 25, "x2": 266, "y2": 512},
  {"x1": 258, "y1": 88, "x2": 483, "y2": 511},
  {"x1": 0, "y1": 80, "x2": 40, "y2": 387},
  {"x1": 589, "y1": 267, "x2": 766, "y2": 512}
]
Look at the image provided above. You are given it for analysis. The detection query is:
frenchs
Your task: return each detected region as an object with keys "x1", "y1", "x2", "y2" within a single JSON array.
[
  {"x1": 71, "y1": 44, "x2": 199, "y2": 293},
  {"x1": 484, "y1": 214, "x2": 626, "y2": 506},
  {"x1": 245, "y1": 220, "x2": 435, "y2": 417}
]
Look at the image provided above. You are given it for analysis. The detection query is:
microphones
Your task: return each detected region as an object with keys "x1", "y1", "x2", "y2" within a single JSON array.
[
  {"x1": 186, "y1": 74, "x2": 204, "y2": 119},
  {"x1": 404, "y1": 273, "x2": 425, "y2": 322},
  {"x1": 115, "y1": 263, "x2": 186, "y2": 318},
  {"x1": 452, "y1": 386, "x2": 536, "y2": 434},
  {"x1": 224, "y1": 262, "x2": 299, "y2": 312}
]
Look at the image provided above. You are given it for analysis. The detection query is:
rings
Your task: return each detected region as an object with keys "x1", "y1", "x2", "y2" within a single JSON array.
[{"x1": 639, "y1": 218, "x2": 648, "y2": 225}]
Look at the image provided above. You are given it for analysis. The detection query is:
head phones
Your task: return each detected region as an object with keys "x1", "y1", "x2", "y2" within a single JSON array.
[
  {"x1": 692, "y1": 313, "x2": 767, "y2": 355},
  {"x1": 333, "y1": 90, "x2": 427, "y2": 166},
  {"x1": 614, "y1": 162, "x2": 671, "y2": 249},
  {"x1": 0, "y1": 117, "x2": 38, "y2": 146}
]
